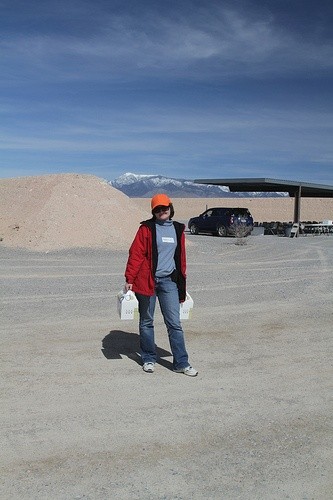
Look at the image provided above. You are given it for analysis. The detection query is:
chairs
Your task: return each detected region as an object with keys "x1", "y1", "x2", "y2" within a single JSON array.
[{"x1": 254, "y1": 220, "x2": 333, "y2": 237}]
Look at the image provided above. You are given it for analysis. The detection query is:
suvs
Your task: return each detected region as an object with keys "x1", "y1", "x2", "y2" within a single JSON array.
[{"x1": 188, "y1": 207, "x2": 254, "y2": 237}]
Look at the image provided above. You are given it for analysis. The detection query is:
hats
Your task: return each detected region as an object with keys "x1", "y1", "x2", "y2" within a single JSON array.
[{"x1": 151, "y1": 194, "x2": 172, "y2": 210}]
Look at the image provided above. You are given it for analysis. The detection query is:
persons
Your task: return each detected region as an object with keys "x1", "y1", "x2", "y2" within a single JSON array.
[{"x1": 125, "y1": 194, "x2": 198, "y2": 376}]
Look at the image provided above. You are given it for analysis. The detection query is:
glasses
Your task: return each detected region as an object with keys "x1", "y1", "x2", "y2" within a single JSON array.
[{"x1": 152, "y1": 205, "x2": 170, "y2": 213}]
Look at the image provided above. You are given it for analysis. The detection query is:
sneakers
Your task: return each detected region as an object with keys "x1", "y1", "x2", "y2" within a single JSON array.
[
  {"x1": 173, "y1": 365, "x2": 198, "y2": 377},
  {"x1": 143, "y1": 362, "x2": 154, "y2": 372}
]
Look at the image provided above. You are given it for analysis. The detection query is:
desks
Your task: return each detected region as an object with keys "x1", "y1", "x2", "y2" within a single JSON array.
[{"x1": 300, "y1": 224, "x2": 333, "y2": 231}]
